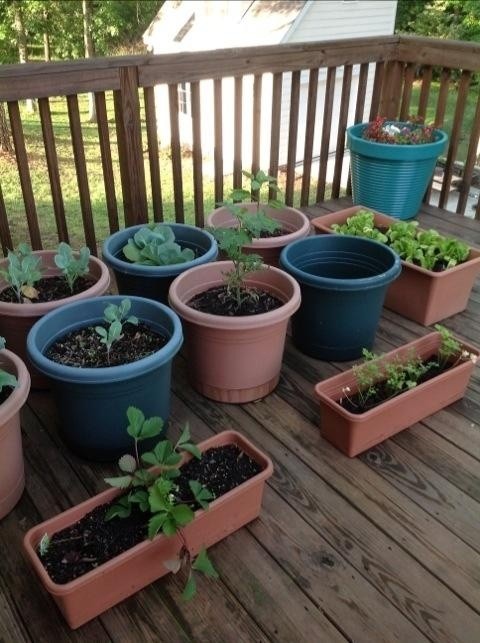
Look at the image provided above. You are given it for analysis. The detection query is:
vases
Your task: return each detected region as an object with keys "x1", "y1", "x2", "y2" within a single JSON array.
[
  {"x1": 347, "y1": 121, "x2": 449, "y2": 220},
  {"x1": 278, "y1": 234, "x2": 402, "y2": 362}
]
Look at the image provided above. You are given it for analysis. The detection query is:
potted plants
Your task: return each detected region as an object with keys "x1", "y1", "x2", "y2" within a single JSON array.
[
  {"x1": 0, "y1": 241, "x2": 110, "y2": 389},
  {"x1": 314, "y1": 323, "x2": 480, "y2": 458},
  {"x1": 206, "y1": 170, "x2": 311, "y2": 269},
  {"x1": 26, "y1": 295, "x2": 185, "y2": 461},
  {"x1": 22, "y1": 405, "x2": 274, "y2": 630},
  {"x1": 0, "y1": 336, "x2": 30, "y2": 519},
  {"x1": 103, "y1": 222, "x2": 219, "y2": 326},
  {"x1": 311, "y1": 204, "x2": 480, "y2": 328},
  {"x1": 167, "y1": 202, "x2": 302, "y2": 404}
]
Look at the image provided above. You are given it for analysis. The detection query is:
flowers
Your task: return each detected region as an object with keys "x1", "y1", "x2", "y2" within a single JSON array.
[{"x1": 361, "y1": 115, "x2": 443, "y2": 144}]
